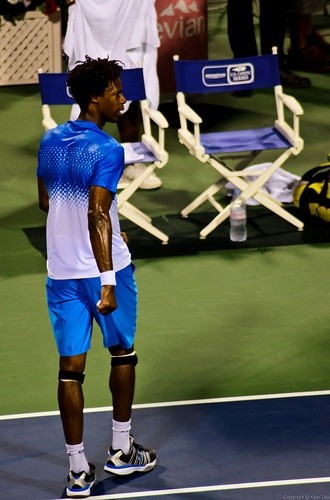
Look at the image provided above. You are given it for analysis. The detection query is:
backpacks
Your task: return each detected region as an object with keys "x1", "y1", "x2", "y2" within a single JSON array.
[{"x1": 292, "y1": 153, "x2": 330, "y2": 228}]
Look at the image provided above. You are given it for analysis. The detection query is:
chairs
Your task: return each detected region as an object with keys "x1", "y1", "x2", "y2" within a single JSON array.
[
  {"x1": 173, "y1": 46, "x2": 304, "y2": 240},
  {"x1": 39, "y1": 63, "x2": 170, "y2": 245}
]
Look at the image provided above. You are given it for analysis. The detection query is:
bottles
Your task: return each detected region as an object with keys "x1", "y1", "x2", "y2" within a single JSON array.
[{"x1": 230, "y1": 189, "x2": 248, "y2": 241}]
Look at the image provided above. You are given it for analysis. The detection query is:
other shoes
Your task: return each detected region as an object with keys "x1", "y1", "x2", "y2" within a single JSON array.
[
  {"x1": 117, "y1": 163, "x2": 163, "y2": 191},
  {"x1": 280, "y1": 64, "x2": 312, "y2": 89},
  {"x1": 231, "y1": 88, "x2": 252, "y2": 98}
]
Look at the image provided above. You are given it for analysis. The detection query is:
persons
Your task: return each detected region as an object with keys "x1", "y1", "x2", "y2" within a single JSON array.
[
  {"x1": 36, "y1": 54, "x2": 158, "y2": 497},
  {"x1": 224, "y1": 0, "x2": 311, "y2": 97},
  {"x1": 61, "y1": 0, "x2": 163, "y2": 189}
]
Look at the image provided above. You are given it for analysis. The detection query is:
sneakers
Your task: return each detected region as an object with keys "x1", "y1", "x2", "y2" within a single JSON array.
[
  {"x1": 103, "y1": 432, "x2": 158, "y2": 476},
  {"x1": 65, "y1": 462, "x2": 98, "y2": 497}
]
